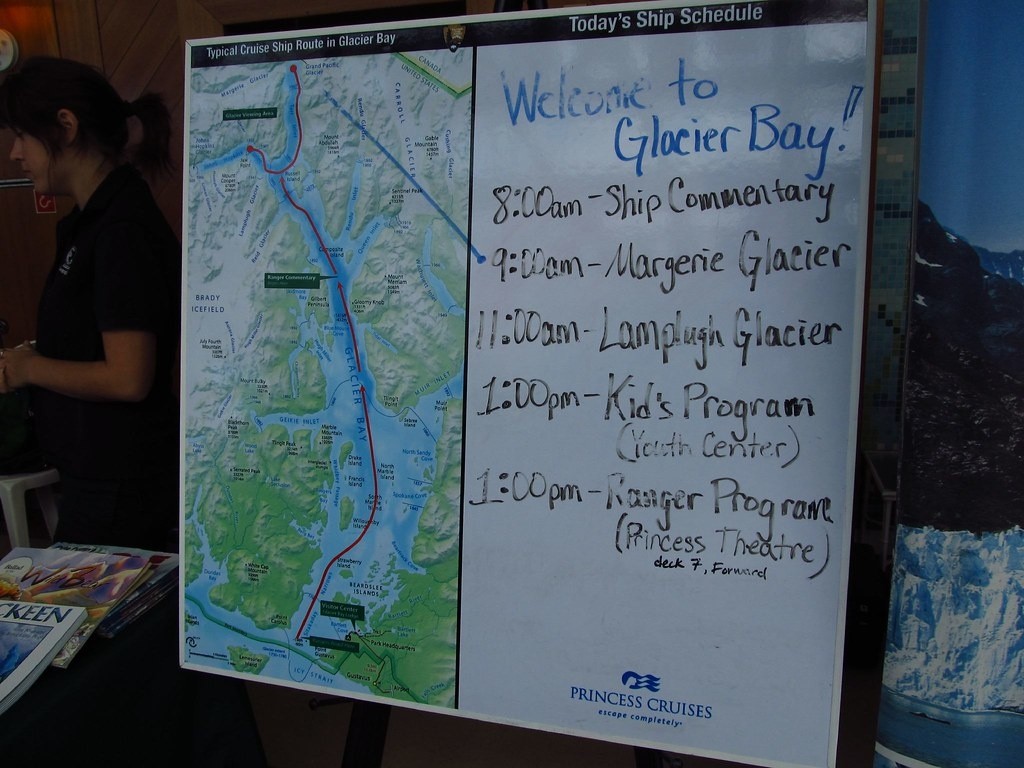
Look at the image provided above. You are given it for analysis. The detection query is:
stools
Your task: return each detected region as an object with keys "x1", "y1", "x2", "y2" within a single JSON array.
[{"x1": 0, "y1": 467, "x2": 61, "y2": 557}]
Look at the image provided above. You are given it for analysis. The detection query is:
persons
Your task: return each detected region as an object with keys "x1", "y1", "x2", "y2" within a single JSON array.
[{"x1": 0, "y1": 55, "x2": 182, "y2": 546}]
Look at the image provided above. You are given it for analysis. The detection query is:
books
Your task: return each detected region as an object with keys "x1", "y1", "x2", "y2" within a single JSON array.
[{"x1": 0, "y1": 542, "x2": 180, "y2": 713}]
